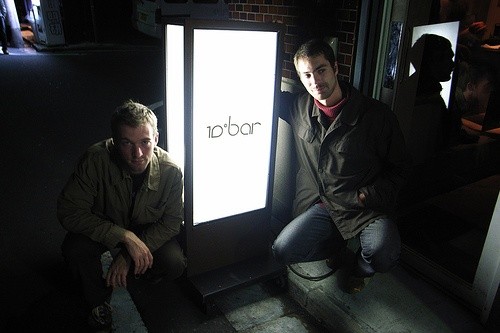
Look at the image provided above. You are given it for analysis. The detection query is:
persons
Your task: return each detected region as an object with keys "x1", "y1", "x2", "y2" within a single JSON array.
[
  {"x1": 271, "y1": 38, "x2": 404, "y2": 290},
  {"x1": 56, "y1": 99, "x2": 192, "y2": 329},
  {"x1": 403, "y1": 33, "x2": 498, "y2": 193},
  {"x1": 0, "y1": 6, "x2": 10, "y2": 55}
]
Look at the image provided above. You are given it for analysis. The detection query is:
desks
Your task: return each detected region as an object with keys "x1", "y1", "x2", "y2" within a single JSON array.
[{"x1": 457, "y1": 43, "x2": 500, "y2": 131}]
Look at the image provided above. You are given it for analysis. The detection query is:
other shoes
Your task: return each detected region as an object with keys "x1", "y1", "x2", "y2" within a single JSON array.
[
  {"x1": 325, "y1": 239, "x2": 347, "y2": 267},
  {"x1": 352, "y1": 247, "x2": 374, "y2": 277}
]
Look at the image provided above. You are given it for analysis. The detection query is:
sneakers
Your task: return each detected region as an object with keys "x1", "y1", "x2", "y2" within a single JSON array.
[{"x1": 92, "y1": 298, "x2": 112, "y2": 332}]
